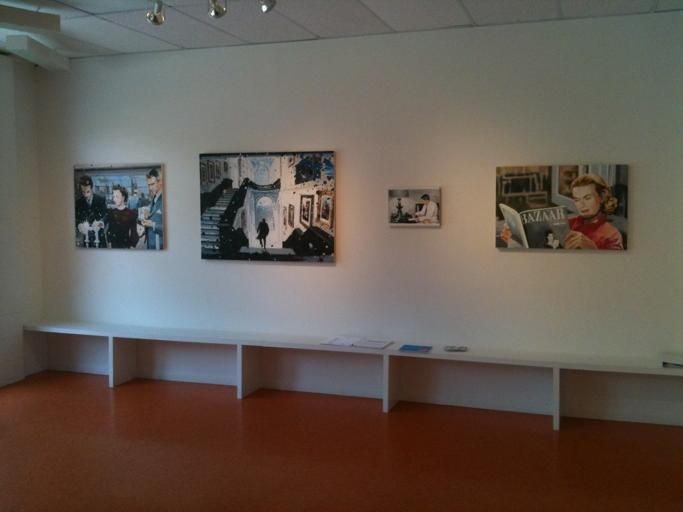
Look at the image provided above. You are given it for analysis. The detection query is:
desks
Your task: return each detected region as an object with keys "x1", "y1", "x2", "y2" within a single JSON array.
[{"x1": 23, "y1": 324, "x2": 683, "y2": 434}]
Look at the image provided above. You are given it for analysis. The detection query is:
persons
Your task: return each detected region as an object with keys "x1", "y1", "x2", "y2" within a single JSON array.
[
  {"x1": 413, "y1": 194, "x2": 438, "y2": 222},
  {"x1": 106, "y1": 184, "x2": 139, "y2": 248},
  {"x1": 142, "y1": 169, "x2": 164, "y2": 249},
  {"x1": 255, "y1": 218, "x2": 269, "y2": 252},
  {"x1": 495, "y1": 173, "x2": 624, "y2": 250},
  {"x1": 74, "y1": 176, "x2": 106, "y2": 247}
]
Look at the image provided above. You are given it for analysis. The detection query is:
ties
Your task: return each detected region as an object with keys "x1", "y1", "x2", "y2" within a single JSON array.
[{"x1": 152, "y1": 194, "x2": 156, "y2": 205}]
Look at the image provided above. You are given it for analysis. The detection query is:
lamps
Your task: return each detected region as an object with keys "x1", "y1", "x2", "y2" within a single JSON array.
[
  {"x1": 0, "y1": 2, "x2": 70, "y2": 73},
  {"x1": 144, "y1": 1, "x2": 279, "y2": 25}
]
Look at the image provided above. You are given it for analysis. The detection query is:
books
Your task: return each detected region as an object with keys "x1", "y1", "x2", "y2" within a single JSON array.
[{"x1": 498, "y1": 201, "x2": 570, "y2": 251}]
